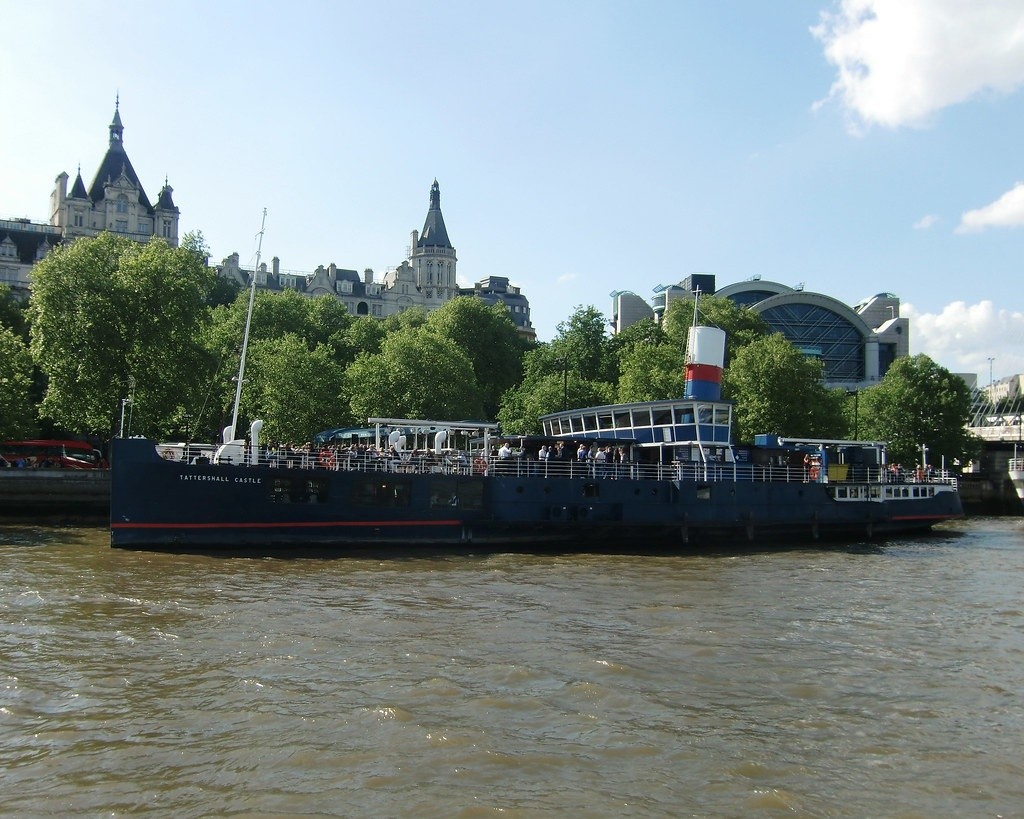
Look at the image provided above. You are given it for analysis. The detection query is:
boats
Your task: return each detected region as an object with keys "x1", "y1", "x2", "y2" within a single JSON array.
[{"x1": 110, "y1": 327, "x2": 963, "y2": 554}]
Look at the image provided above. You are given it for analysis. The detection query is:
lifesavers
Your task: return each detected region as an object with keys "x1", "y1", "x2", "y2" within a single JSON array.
[
  {"x1": 163, "y1": 449, "x2": 176, "y2": 462},
  {"x1": 917, "y1": 469, "x2": 924, "y2": 480},
  {"x1": 475, "y1": 458, "x2": 487, "y2": 472},
  {"x1": 318, "y1": 451, "x2": 336, "y2": 466},
  {"x1": 810, "y1": 466, "x2": 819, "y2": 480}
]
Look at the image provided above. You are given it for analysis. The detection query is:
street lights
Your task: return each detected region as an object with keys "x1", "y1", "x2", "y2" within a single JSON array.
[
  {"x1": 987, "y1": 357, "x2": 996, "y2": 404},
  {"x1": 843, "y1": 389, "x2": 860, "y2": 441},
  {"x1": 554, "y1": 356, "x2": 568, "y2": 410}
]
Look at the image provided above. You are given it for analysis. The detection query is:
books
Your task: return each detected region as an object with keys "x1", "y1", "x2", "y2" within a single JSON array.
[{"x1": 489, "y1": 441, "x2": 629, "y2": 480}]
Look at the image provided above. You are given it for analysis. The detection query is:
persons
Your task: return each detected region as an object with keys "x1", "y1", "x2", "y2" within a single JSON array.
[
  {"x1": 886, "y1": 463, "x2": 934, "y2": 484},
  {"x1": 267, "y1": 439, "x2": 402, "y2": 473},
  {"x1": 16, "y1": 456, "x2": 65, "y2": 469},
  {"x1": 409, "y1": 448, "x2": 469, "y2": 476},
  {"x1": 803, "y1": 454, "x2": 810, "y2": 483}
]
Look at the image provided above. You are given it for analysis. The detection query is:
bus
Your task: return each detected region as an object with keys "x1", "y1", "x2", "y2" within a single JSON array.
[{"x1": 0, "y1": 440, "x2": 110, "y2": 470}]
